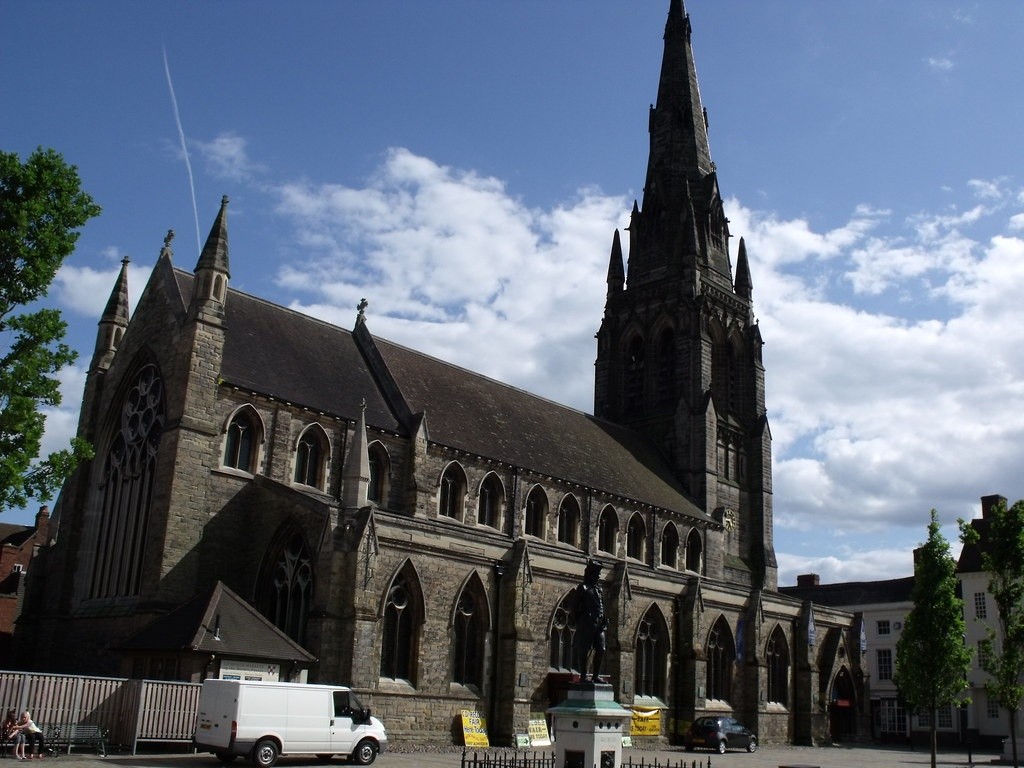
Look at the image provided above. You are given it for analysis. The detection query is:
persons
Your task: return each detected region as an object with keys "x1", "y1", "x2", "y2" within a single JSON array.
[
  {"x1": 7, "y1": 711, "x2": 44, "y2": 759},
  {"x1": 3, "y1": 711, "x2": 26, "y2": 760},
  {"x1": 568, "y1": 558, "x2": 610, "y2": 684}
]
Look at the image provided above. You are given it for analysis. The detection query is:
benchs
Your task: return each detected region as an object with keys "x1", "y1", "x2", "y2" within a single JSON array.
[{"x1": 0, "y1": 721, "x2": 110, "y2": 758}]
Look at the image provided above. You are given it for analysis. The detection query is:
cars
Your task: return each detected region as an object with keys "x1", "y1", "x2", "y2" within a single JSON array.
[{"x1": 685, "y1": 716, "x2": 758, "y2": 754}]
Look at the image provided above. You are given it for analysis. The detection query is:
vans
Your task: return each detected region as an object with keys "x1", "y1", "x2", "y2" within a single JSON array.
[{"x1": 190, "y1": 678, "x2": 389, "y2": 768}]
